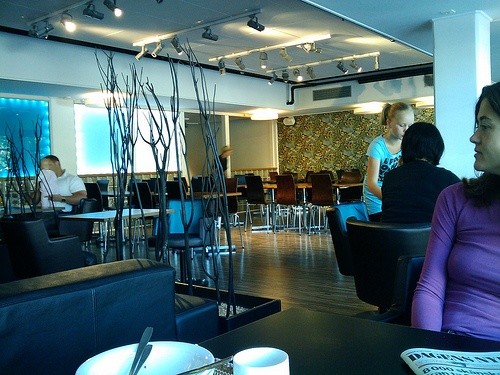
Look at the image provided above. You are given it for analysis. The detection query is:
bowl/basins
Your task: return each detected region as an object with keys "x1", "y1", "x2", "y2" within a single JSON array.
[{"x1": 75, "y1": 341, "x2": 215, "y2": 375}]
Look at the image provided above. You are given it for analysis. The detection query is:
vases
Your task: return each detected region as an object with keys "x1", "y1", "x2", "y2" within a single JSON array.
[{"x1": 175, "y1": 281, "x2": 281, "y2": 334}]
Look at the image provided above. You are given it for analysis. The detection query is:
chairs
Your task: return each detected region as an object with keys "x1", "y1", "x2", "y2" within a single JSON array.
[{"x1": 0, "y1": 168, "x2": 432, "y2": 375}]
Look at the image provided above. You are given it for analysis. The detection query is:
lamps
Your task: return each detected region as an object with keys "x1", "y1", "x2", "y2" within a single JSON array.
[
  {"x1": 354, "y1": 106, "x2": 382, "y2": 114},
  {"x1": 151, "y1": 40, "x2": 165, "y2": 58},
  {"x1": 135, "y1": 44, "x2": 149, "y2": 60},
  {"x1": 170, "y1": 36, "x2": 182, "y2": 53},
  {"x1": 251, "y1": 113, "x2": 278, "y2": 120},
  {"x1": 83, "y1": 1, "x2": 104, "y2": 20},
  {"x1": 218, "y1": 59, "x2": 225, "y2": 74},
  {"x1": 261, "y1": 43, "x2": 323, "y2": 85},
  {"x1": 202, "y1": 26, "x2": 219, "y2": 41},
  {"x1": 235, "y1": 55, "x2": 244, "y2": 70},
  {"x1": 247, "y1": 14, "x2": 265, "y2": 31},
  {"x1": 337, "y1": 60, "x2": 362, "y2": 74},
  {"x1": 103, "y1": 0, "x2": 122, "y2": 17},
  {"x1": 28, "y1": 10, "x2": 76, "y2": 39}
]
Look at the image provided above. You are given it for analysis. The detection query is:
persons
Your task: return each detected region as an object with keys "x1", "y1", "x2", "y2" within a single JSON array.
[
  {"x1": 381, "y1": 122, "x2": 461, "y2": 227},
  {"x1": 411, "y1": 81, "x2": 500, "y2": 341},
  {"x1": 363, "y1": 102, "x2": 415, "y2": 223},
  {"x1": 211, "y1": 146, "x2": 232, "y2": 178},
  {"x1": 28, "y1": 155, "x2": 87, "y2": 216}
]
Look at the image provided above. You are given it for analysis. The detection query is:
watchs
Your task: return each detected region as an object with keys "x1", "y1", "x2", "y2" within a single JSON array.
[{"x1": 61, "y1": 196, "x2": 66, "y2": 202}]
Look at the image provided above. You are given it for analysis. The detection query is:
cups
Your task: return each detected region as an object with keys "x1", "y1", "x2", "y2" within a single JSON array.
[
  {"x1": 42, "y1": 197, "x2": 49, "y2": 210},
  {"x1": 233, "y1": 347, "x2": 290, "y2": 375}
]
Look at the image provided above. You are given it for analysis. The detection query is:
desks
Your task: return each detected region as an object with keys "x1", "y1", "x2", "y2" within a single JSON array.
[
  {"x1": 198, "y1": 307, "x2": 500, "y2": 375},
  {"x1": 102, "y1": 191, "x2": 241, "y2": 253},
  {"x1": 58, "y1": 208, "x2": 175, "y2": 263},
  {"x1": 238, "y1": 184, "x2": 363, "y2": 231}
]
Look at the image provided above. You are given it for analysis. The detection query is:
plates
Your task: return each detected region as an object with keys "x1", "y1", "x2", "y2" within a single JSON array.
[{"x1": 15, "y1": 205, "x2": 34, "y2": 208}]
